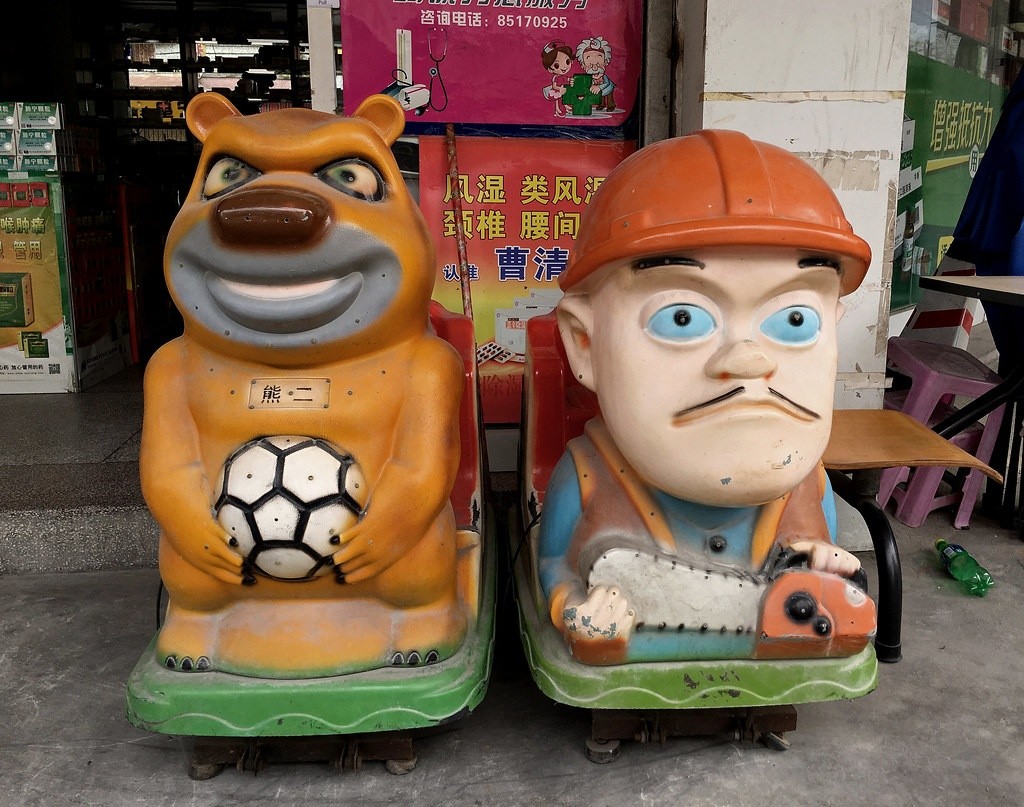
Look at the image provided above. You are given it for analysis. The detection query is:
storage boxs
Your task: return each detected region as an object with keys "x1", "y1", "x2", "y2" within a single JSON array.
[{"x1": 0, "y1": 101, "x2": 94, "y2": 172}]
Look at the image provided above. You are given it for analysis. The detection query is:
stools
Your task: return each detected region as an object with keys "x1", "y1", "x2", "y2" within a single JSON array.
[{"x1": 873, "y1": 337, "x2": 1008, "y2": 529}]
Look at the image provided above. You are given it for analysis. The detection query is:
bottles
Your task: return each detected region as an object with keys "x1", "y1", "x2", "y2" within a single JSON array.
[
  {"x1": 934, "y1": 538, "x2": 995, "y2": 599},
  {"x1": 902, "y1": 205, "x2": 915, "y2": 279}
]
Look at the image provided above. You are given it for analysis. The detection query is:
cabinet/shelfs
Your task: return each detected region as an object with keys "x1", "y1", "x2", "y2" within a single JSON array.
[{"x1": 35, "y1": 26, "x2": 344, "y2": 129}]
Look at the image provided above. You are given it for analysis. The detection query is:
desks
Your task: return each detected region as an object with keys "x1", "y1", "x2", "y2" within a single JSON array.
[
  {"x1": 919, "y1": 275, "x2": 1024, "y2": 533},
  {"x1": 822, "y1": 409, "x2": 1004, "y2": 485}
]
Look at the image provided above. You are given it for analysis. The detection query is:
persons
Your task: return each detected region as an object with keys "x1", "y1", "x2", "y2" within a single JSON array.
[{"x1": 945, "y1": 61, "x2": 1023, "y2": 376}]
